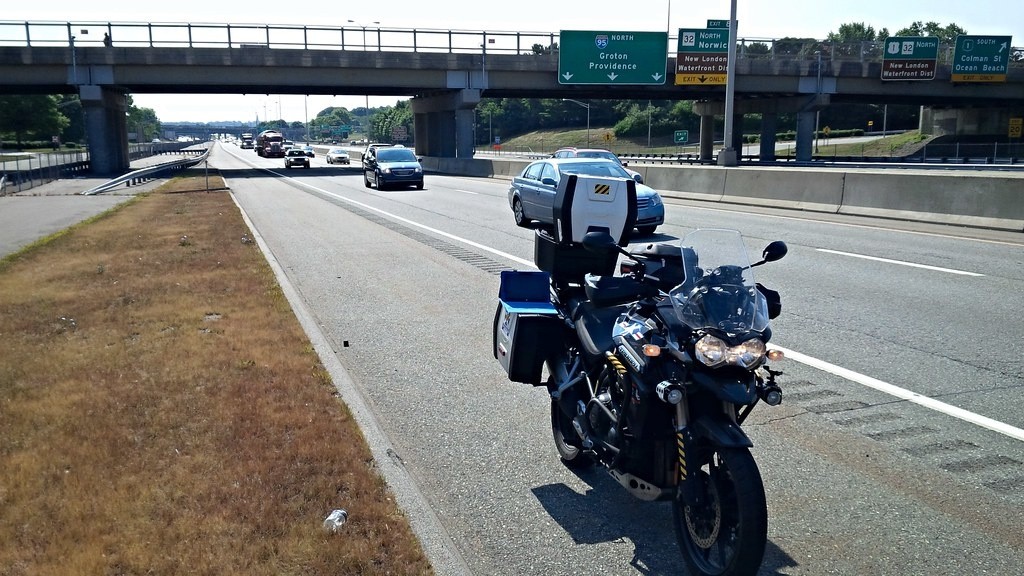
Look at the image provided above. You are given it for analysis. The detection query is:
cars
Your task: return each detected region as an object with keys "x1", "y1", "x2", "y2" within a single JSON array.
[
  {"x1": 508, "y1": 158, "x2": 665, "y2": 235},
  {"x1": 363, "y1": 148, "x2": 425, "y2": 189},
  {"x1": 361, "y1": 143, "x2": 391, "y2": 160},
  {"x1": 326, "y1": 148, "x2": 351, "y2": 164},
  {"x1": 152, "y1": 139, "x2": 162, "y2": 143}
]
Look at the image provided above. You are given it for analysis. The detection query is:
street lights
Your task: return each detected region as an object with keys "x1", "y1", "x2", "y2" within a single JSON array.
[
  {"x1": 348, "y1": 19, "x2": 380, "y2": 51},
  {"x1": 562, "y1": 98, "x2": 589, "y2": 149}
]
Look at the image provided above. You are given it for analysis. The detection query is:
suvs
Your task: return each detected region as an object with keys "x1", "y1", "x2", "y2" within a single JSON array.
[
  {"x1": 284, "y1": 149, "x2": 310, "y2": 169},
  {"x1": 303, "y1": 147, "x2": 315, "y2": 158},
  {"x1": 550, "y1": 147, "x2": 643, "y2": 183},
  {"x1": 283, "y1": 141, "x2": 296, "y2": 153}
]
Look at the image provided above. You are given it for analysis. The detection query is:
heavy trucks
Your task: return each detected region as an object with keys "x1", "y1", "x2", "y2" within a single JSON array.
[
  {"x1": 255, "y1": 130, "x2": 287, "y2": 158},
  {"x1": 240, "y1": 134, "x2": 254, "y2": 148}
]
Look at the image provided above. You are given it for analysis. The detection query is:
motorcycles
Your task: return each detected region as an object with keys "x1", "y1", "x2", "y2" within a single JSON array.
[{"x1": 491, "y1": 173, "x2": 790, "y2": 576}]
